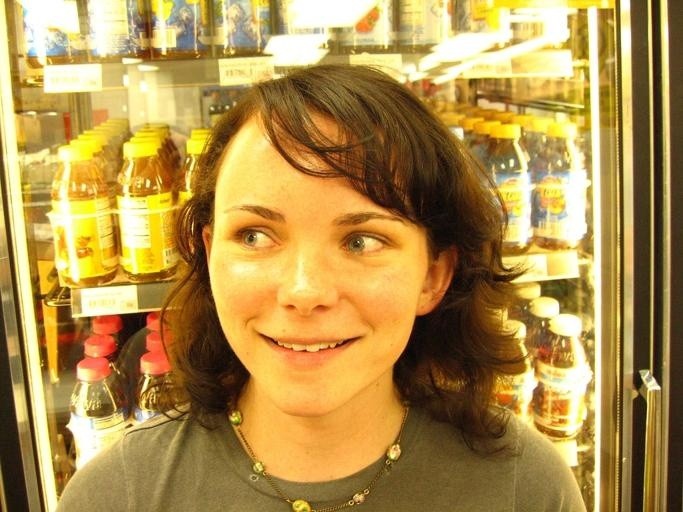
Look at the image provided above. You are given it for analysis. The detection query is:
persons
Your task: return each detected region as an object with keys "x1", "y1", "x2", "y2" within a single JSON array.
[{"x1": 52, "y1": 58, "x2": 590, "y2": 512}]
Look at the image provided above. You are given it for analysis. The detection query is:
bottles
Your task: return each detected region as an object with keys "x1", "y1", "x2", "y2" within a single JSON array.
[
  {"x1": 20, "y1": 0, "x2": 575, "y2": 66},
  {"x1": 48, "y1": 115, "x2": 212, "y2": 476},
  {"x1": 414, "y1": 95, "x2": 588, "y2": 441}
]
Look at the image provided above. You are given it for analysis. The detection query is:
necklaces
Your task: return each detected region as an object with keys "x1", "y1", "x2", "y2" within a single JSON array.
[{"x1": 221, "y1": 374, "x2": 412, "y2": 512}]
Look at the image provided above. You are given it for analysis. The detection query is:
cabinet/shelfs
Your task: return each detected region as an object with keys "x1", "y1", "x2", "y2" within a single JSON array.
[{"x1": 0, "y1": 0, "x2": 623, "y2": 512}]
